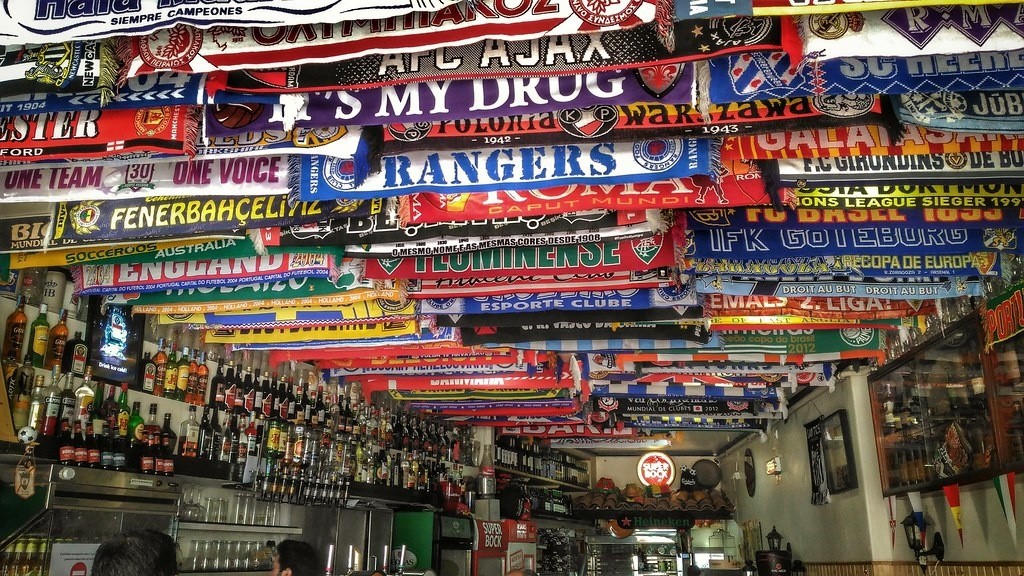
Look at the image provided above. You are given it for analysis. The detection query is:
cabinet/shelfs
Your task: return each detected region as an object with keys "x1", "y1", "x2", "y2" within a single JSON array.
[
  {"x1": 0, "y1": 294, "x2": 595, "y2": 576},
  {"x1": 866, "y1": 283, "x2": 1024, "y2": 500}
]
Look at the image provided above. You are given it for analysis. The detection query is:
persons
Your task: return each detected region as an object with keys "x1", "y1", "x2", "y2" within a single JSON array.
[
  {"x1": 90, "y1": 529, "x2": 179, "y2": 576},
  {"x1": 269, "y1": 539, "x2": 320, "y2": 576}
]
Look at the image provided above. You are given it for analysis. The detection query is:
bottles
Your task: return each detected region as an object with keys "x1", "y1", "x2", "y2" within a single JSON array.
[
  {"x1": 0, "y1": 263, "x2": 591, "y2": 576},
  {"x1": 879, "y1": 332, "x2": 1024, "y2": 491}
]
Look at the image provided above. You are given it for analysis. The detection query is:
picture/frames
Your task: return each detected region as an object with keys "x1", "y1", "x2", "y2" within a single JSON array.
[{"x1": 821, "y1": 408, "x2": 859, "y2": 494}]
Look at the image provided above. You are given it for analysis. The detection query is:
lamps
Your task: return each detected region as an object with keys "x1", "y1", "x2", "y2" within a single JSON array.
[
  {"x1": 766, "y1": 524, "x2": 785, "y2": 551},
  {"x1": 741, "y1": 560, "x2": 757, "y2": 576},
  {"x1": 899, "y1": 509, "x2": 945, "y2": 575}
]
